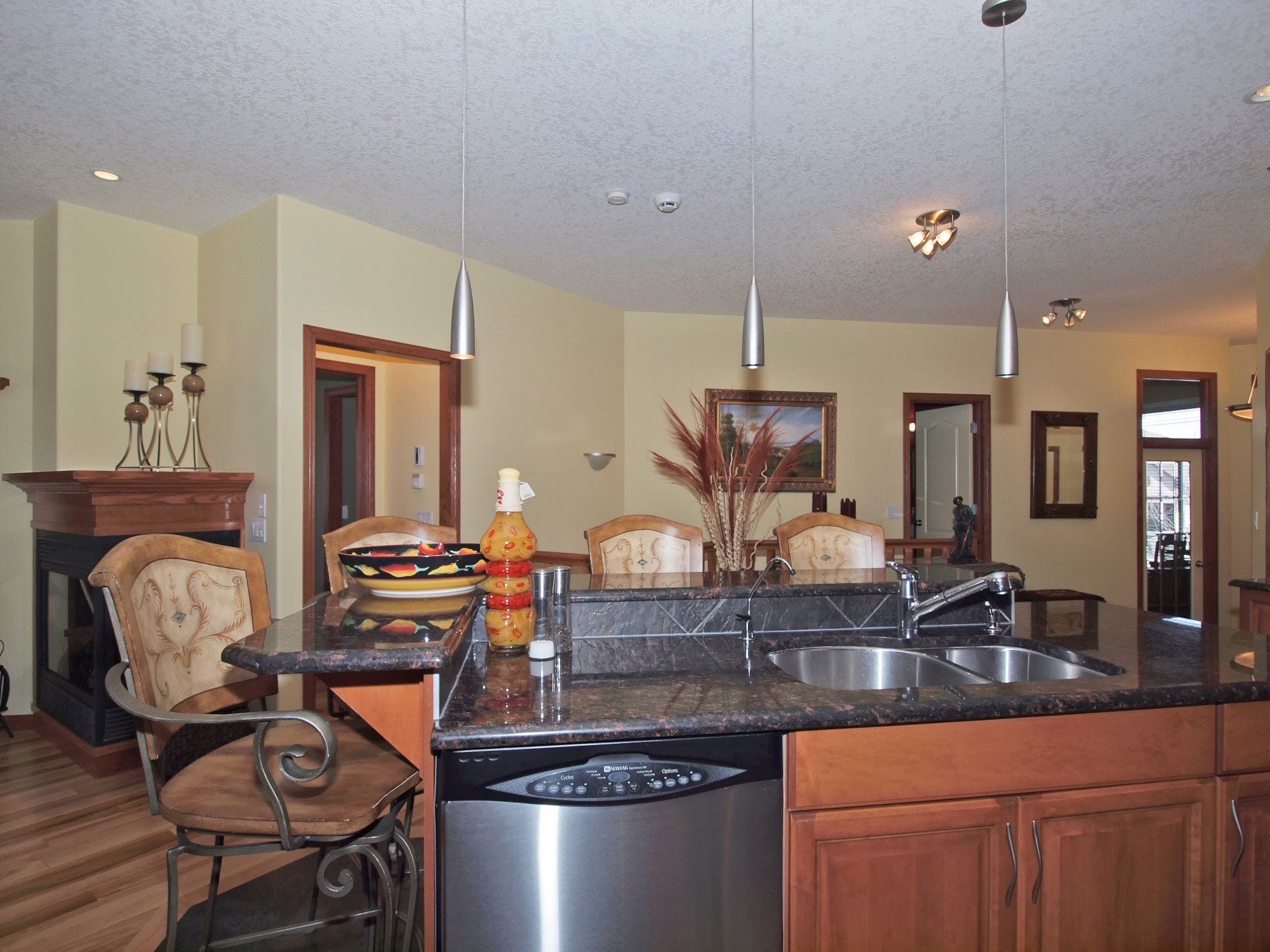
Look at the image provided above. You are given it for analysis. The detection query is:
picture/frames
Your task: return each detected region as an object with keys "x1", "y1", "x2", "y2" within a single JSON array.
[{"x1": 705, "y1": 388, "x2": 839, "y2": 493}]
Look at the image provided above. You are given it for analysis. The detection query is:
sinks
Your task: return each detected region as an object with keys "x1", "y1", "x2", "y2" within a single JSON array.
[
  {"x1": 909, "y1": 645, "x2": 1111, "y2": 685},
  {"x1": 758, "y1": 645, "x2": 997, "y2": 692}
]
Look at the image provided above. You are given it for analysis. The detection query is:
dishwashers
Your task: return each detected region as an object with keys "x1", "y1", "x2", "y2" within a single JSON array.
[{"x1": 434, "y1": 732, "x2": 782, "y2": 951}]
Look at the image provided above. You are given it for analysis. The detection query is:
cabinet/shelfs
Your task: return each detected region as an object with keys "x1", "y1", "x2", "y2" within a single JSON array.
[
  {"x1": 780, "y1": 693, "x2": 1223, "y2": 952},
  {"x1": 1210, "y1": 696, "x2": 1269, "y2": 952}
]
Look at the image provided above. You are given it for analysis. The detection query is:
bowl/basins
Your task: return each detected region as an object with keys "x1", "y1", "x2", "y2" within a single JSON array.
[{"x1": 338, "y1": 543, "x2": 489, "y2": 598}]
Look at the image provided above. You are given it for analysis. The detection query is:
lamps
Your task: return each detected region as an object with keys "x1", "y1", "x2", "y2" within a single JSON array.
[
  {"x1": 584, "y1": 453, "x2": 616, "y2": 471},
  {"x1": 908, "y1": 210, "x2": 963, "y2": 256},
  {"x1": 1041, "y1": 295, "x2": 1087, "y2": 327},
  {"x1": 981, "y1": 0, "x2": 1026, "y2": 379},
  {"x1": 449, "y1": 0, "x2": 478, "y2": 360},
  {"x1": 740, "y1": 0, "x2": 765, "y2": 369}
]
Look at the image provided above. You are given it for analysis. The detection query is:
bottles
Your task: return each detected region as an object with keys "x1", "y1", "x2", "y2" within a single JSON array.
[{"x1": 480, "y1": 467, "x2": 539, "y2": 655}]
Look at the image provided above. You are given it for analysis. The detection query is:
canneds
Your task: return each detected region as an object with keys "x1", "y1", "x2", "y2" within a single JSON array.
[{"x1": 480, "y1": 469, "x2": 538, "y2": 657}]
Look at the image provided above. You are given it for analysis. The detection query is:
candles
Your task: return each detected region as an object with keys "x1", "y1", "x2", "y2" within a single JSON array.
[
  {"x1": 182, "y1": 322, "x2": 206, "y2": 364},
  {"x1": 123, "y1": 357, "x2": 149, "y2": 393},
  {"x1": 147, "y1": 350, "x2": 174, "y2": 374}
]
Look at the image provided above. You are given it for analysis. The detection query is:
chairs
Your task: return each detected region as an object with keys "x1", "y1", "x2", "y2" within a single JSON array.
[
  {"x1": 88, "y1": 531, "x2": 420, "y2": 952},
  {"x1": 321, "y1": 512, "x2": 461, "y2": 600},
  {"x1": 582, "y1": 513, "x2": 704, "y2": 575},
  {"x1": 771, "y1": 512, "x2": 886, "y2": 571}
]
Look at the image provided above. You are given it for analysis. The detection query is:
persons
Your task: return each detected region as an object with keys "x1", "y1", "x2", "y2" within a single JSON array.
[{"x1": 946, "y1": 496, "x2": 979, "y2": 565}]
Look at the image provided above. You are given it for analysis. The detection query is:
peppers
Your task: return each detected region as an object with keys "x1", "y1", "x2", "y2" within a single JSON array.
[{"x1": 417, "y1": 541, "x2": 444, "y2": 555}]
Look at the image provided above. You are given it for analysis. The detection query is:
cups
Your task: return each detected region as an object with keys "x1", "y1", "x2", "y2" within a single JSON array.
[
  {"x1": 548, "y1": 565, "x2": 572, "y2": 595},
  {"x1": 531, "y1": 569, "x2": 554, "y2": 599}
]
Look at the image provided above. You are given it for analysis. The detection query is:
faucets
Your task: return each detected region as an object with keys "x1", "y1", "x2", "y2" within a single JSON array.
[
  {"x1": 735, "y1": 556, "x2": 796, "y2": 642},
  {"x1": 885, "y1": 559, "x2": 1011, "y2": 637}
]
